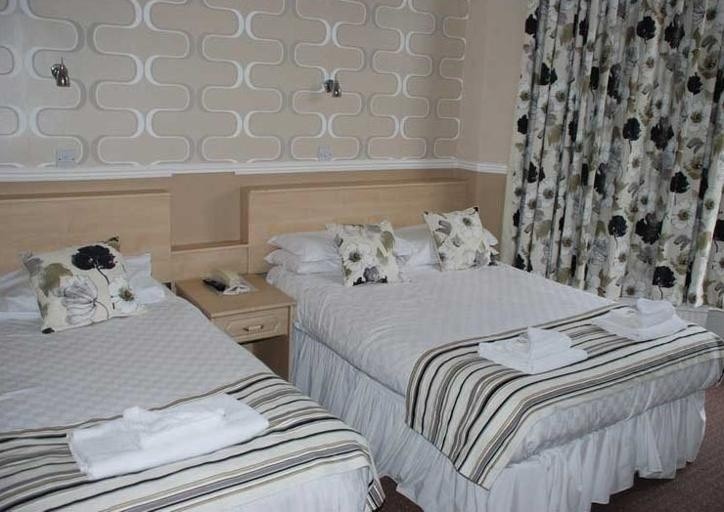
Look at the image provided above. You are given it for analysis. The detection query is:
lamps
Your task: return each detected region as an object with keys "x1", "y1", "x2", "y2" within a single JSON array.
[
  {"x1": 50, "y1": 57, "x2": 72, "y2": 87},
  {"x1": 325, "y1": 77, "x2": 343, "y2": 96}
]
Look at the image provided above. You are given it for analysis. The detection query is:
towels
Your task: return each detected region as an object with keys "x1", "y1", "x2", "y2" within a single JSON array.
[
  {"x1": 610, "y1": 299, "x2": 674, "y2": 327},
  {"x1": 504, "y1": 325, "x2": 572, "y2": 359},
  {"x1": 593, "y1": 313, "x2": 688, "y2": 343},
  {"x1": 66, "y1": 392, "x2": 268, "y2": 484},
  {"x1": 123, "y1": 404, "x2": 224, "y2": 448},
  {"x1": 478, "y1": 339, "x2": 588, "y2": 375}
]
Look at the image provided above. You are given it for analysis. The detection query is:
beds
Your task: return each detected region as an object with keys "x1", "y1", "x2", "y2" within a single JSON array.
[
  {"x1": 0, "y1": 284, "x2": 385, "y2": 512},
  {"x1": 266, "y1": 262, "x2": 724, "y2": 512}
]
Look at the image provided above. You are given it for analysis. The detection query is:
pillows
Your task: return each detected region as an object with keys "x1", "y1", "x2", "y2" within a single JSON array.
[
  {"x1": 266, "y1": 228, "x2": 414, "y2": 262},
  {"x1": 263, "y1": 250, "x2": 344, "y2": 275},
  {"x1": 396, "y1": 220, "x2": 498, "y2": 258},
  {"x1": 0, "y1": 250, "x2": 165, "y2": 318},
  {"x1": 416, "y1": 247, "x2": 499, "y2": 265},
  {"x1": 18, "y1": 234, "x2": 147, "y2": 335},
  {"x1": 326, "y1": 218, "x2": 415, "y2": 289},
  {"x1": 420, "y1": 203, "x2": 499, "y2": 273}
]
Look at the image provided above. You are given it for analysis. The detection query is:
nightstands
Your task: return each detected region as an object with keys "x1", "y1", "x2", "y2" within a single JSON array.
[{"x1": 175, "y1": 274, "x2": 297, "y2": 386}]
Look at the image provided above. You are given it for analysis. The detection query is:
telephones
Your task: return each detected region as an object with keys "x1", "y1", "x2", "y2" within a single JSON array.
[{"x1": 216, "y1": 265, "x2": 240, "y2": 286}]
